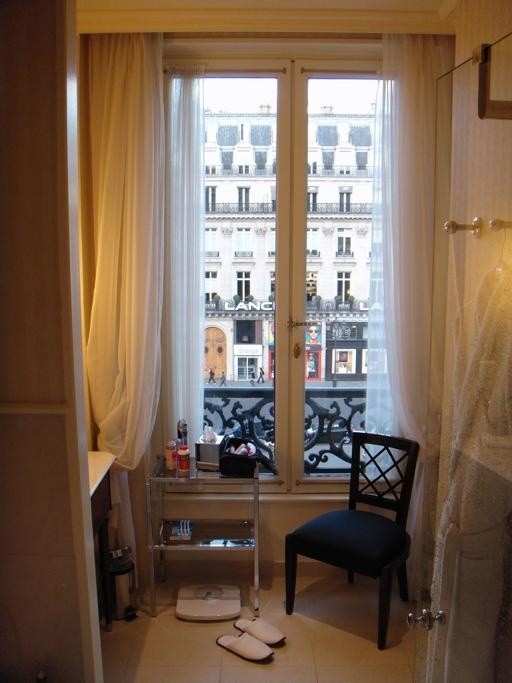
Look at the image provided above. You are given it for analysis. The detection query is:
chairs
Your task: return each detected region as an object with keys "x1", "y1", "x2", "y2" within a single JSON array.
[{"x1": 285, "y1": 432, "x2": 419, "y2": 650}]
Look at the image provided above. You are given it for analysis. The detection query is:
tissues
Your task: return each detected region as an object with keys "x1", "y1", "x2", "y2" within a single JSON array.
[{"x1": 195, "y1": 425, "x2": 224, "y2": 463}]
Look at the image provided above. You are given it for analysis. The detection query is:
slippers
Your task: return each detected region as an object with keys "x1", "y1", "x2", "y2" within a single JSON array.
[
  {"x1": 216, "y1": 632, "x2": 275, "y2": 661},
  {"x1": 233, "y1": 616, "x2": 286, "y2": 647}
]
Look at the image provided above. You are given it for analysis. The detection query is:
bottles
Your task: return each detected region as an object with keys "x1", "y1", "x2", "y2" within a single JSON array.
[{"x1": 165, "y1": 418, "x2": 190, "y2": 473}]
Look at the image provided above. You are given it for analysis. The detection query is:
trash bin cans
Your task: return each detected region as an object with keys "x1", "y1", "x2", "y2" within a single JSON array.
[{"x1": 104, "y1": 546, "x2": 137, "y2": 621}]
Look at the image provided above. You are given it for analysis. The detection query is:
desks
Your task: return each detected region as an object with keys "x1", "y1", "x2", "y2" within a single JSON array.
[{"x1": 88, "y1": 451, "x2": 117, "y2": 631}]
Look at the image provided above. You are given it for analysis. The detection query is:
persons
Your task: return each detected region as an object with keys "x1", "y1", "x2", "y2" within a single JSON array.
[
  {"x1": 218, "y1": 370, "x2": 227, "y2": 387},
  {"x1": 306, "y1": 325, "x2": 322, "y2": 343},
  {"x1": 207, "y1": 368, "x2": 217, "y2": 383},
  {"x1": 256, "y1": 368, "x2": 265, "y2": 382}
]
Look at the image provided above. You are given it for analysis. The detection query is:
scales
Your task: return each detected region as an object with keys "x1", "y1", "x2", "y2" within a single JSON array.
[{"x1": 175, "y1": 584, "x2": 241, "y2": 621}]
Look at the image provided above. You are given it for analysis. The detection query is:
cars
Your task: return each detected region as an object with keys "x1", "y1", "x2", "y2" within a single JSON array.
[{"x1": 226, "y1": 415, "x2": 355, "y2": 445}]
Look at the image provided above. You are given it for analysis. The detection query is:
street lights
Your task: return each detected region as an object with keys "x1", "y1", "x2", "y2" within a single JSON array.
[{"x1": 327, "y1": 320, "x2": 347, "y2": 387}]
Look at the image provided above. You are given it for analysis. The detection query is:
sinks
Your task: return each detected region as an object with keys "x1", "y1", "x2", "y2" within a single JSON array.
[{"x1": 89, "y1": 450, "x2": 116, "y2": 498}]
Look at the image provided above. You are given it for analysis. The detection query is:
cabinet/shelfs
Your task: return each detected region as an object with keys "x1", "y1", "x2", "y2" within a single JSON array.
[{"x1": 144, "y1": 454, "x2": 259, "y2": 617}]
